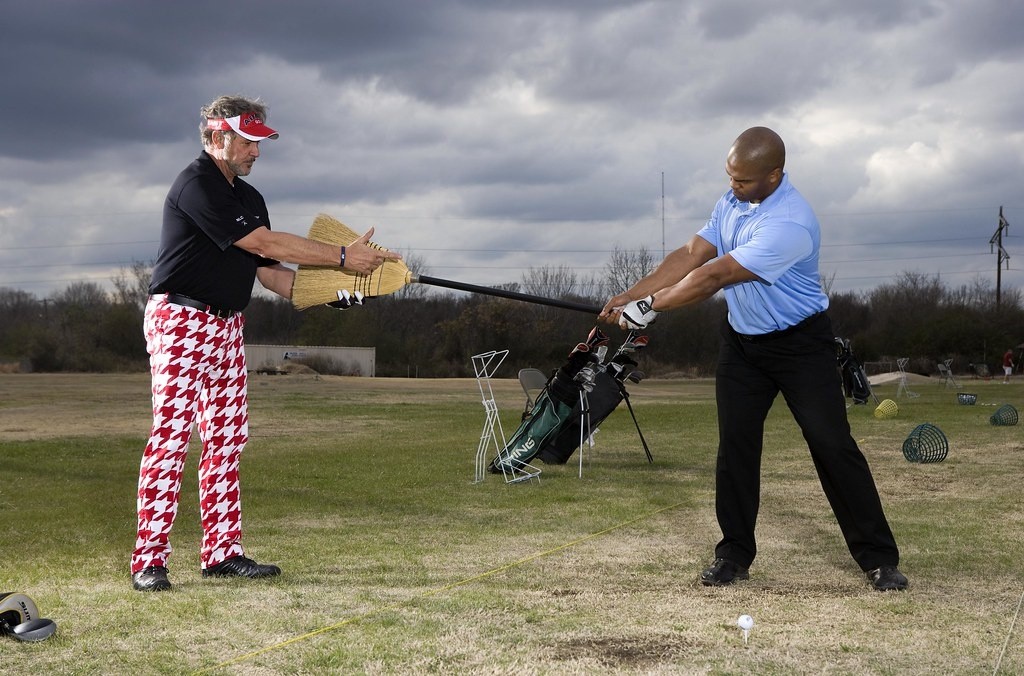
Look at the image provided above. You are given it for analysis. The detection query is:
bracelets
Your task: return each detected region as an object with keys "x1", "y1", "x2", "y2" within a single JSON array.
[{"x1": 340, "y1": 246, "x2": 345, "y2": 268}]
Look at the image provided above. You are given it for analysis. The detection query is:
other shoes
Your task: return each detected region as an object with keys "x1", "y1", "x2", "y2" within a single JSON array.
[{"x1": 1003, "y1": 381, "x2": 1009, "y2": 384}]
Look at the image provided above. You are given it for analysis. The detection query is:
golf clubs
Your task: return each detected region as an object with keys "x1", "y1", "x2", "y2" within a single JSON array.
[
  {"x1": 0, "y1": 614, "x2": 57, "y2": 642},
  {"x1": 835, "y1": 336, "x2": 853, "y2": 365},
  {"x1": 571, "y1": 323, "x2": 655, "y2": 388}
]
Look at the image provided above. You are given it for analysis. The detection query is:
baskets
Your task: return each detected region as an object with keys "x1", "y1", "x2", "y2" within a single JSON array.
[
  {"x1": 902, "y1": 424, "x2": 949, "y2": 463},
  {"x1": 874, "y1": 399, "x2": 899, "y2": 419},
  {"x1": 990, "y1": 404, "x2": 1018, "y2": 426},
  {"x1": 957, "y1": 393, "x2": 977, "y2": 405}
]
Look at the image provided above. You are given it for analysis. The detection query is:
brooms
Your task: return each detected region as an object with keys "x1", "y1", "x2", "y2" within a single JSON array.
[{"x1": 290, "y1": 212, "x2": 656, "y2": 326}]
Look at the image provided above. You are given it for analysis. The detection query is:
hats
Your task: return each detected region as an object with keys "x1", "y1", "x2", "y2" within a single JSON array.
[{"x1": 200, "y1": 112, "x2": 279, "y2": 142}]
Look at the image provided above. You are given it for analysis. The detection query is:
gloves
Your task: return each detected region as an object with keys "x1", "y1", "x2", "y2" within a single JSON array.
[
  {"x1": 325, "y1": 289, "x2": 365, "y2": 311},
  {"x1": 618, "y1": 295, "x2": 662, "y2": 330}
]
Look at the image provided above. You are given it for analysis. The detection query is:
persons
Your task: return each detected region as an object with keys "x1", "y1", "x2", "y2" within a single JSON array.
[
  {"x1": 1003, "y1": 349, "x2": 1015, "y2": 385},
  {"x1": 130, "y1": 95, "x2": 402, "y2": 591},
  {"x1": 597, "y1": 127, "x2": 908, "y2": 590}
]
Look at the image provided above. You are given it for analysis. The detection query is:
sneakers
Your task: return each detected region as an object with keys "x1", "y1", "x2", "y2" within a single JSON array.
[
  {"x1": 866, "y1": 566, "x2": 908, "y2": 592},
  {"x1": 201, "y1": 555, "x2": 281, "y2": 579},
  {"x1": 132, "y1": 566, "x2": 171, "y2": 592},
  {"x1": 701, "y1": 558, "x2": 749, "y2": 587}
]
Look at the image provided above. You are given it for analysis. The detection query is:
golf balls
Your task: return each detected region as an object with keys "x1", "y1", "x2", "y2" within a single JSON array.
[
  {"x1": 959, "y1": 395, "x2": 976, "y2": 406},
  {"x1": 738, "y1": 614, "x2": 755, "y2": 630}
]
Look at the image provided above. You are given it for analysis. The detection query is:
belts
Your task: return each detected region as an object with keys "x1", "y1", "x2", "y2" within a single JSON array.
[
  {"x1": 727, "y1": 312, "x2": 823, "y2": 344},
  {"x1": 147, "y1": 294, "x2": 235, "y2": 319}
]
